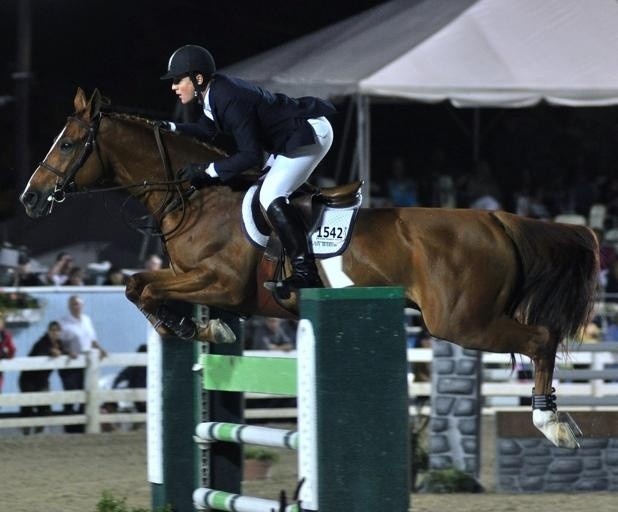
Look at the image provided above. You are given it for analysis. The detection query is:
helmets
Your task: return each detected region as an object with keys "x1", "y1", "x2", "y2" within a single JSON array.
[{"x1": 159, "y1": 44, "x2": 216, "y2": 81}]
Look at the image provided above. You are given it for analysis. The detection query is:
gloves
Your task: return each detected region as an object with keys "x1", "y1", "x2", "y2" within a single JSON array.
[
  {"x1": 177, "y1": 161, "x2": 215, "y2": 182},
  {"x1": 155, "y1": 120, "x2": 170, "y2": 130}
]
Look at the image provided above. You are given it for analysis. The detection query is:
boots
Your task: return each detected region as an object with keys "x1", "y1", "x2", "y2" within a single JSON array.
[{"x1": 263, "y1": 195, "x2": 326, "y2": 298}]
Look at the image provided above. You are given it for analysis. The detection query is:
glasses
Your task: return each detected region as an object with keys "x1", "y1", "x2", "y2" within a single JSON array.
[{"x1": 172, "y1": 74, "x2": 190, "y2": 84}]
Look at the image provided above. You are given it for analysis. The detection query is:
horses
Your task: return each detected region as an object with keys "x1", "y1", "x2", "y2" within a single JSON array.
[{"x1": 19, "y1": 88, "x2": 599, "y2": 449}]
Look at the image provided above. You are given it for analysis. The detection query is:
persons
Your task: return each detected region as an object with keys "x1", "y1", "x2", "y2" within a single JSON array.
[
  {"x1": 157, "y1": 45, "x2": 334, "y2": 292},
  {"x1": 0, "y1": 244, "x2": 163, "y2": 435},
  {"x1": 371, "y1": 153, "x2": 618, "y2": 297}
]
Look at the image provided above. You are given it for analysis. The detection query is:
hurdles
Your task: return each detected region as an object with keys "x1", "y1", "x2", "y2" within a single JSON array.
[{"x1": 147, "y1": 284, "x2": 408, "y2": 512}]
